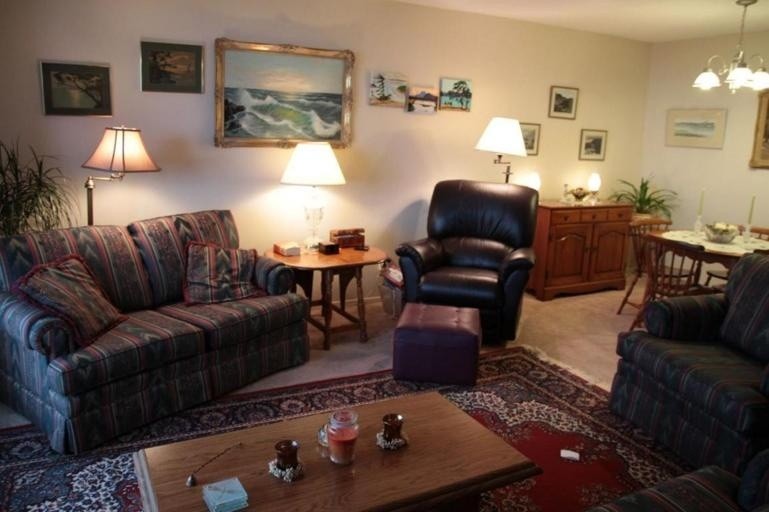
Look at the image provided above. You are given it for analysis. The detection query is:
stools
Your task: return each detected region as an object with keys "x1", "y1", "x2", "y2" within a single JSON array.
[{"x1": 389, "y1": 301, "x2": 485, "y2": 391}]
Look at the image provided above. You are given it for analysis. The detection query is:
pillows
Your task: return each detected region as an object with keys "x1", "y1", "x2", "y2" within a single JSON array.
[
  {"x1": 179, "y1": 235, "x2": 263, "y2": 307},
  {"x1": 9, "y1": 252, "x2": 131, "y2": 353}
]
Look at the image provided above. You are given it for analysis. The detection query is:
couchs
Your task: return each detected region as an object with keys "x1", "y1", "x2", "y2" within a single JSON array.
[
  {"x1": 0, "y1": 207, "x2": 314, "y2": 459},
  {"x1": 571, "y1": 443, "x2": 768, "y2": 511},
  {"x1": 394, "y1": 178, "x2": 543, "y2": 351},
  {"x1": 608, "y1": 250, "x2": 769, "y2": 476}
]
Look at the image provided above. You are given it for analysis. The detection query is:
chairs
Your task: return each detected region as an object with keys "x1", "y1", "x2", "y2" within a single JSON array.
[{"x1": 614, "y1": 212, "x2": 769, "y2": 335}]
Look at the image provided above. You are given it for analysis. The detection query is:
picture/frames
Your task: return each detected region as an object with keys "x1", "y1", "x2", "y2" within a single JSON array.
[
  {"x1": 39, "y1": 58, "x2": 114, "y2": 119},
  {"x1": 579, "y1": 128, "x2": 608, "y2": 162},
  {"x1": 140, "y1": 39, "x2": 206, "y2": 95},
  {"x1": 213, "y1": 37, "x2": 354, "y2": 150},
  {"x1": 548, "y1": 84, "x2": 580, "y2": 120},
  {"x1": 517, "y1": 121, "x2": 542, "y2": 157},
  {"x1": 744, "y1": 87, "x2": 769, "y2": 171},
  {"x1": 664, "y1": 106, "x2": 729, "y2": 150}
]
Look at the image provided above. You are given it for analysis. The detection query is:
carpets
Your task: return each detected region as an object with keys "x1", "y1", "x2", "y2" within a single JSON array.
[{"x1": 0, "y1": 342, "x2": 699, "y2": 512}]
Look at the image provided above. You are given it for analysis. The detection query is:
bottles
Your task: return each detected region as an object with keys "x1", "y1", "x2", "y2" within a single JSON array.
[{"x1": 326, "y1": 411, "x2": 360, "y2": 466}]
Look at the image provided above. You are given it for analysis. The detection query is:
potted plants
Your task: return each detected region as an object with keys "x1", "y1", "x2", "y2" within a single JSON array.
[{"x1": 605, "y1": 175, "x2": 683, "y2": 219}]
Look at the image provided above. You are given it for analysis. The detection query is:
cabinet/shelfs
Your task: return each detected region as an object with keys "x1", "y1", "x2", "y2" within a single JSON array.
[{"x1": 524, "y1": 197, "x2": 638, "y2": 303}]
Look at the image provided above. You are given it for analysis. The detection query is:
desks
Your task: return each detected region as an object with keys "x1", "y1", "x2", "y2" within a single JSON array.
[{"x1": 265, "y1": 240, "x2": 391, "y2": 352}]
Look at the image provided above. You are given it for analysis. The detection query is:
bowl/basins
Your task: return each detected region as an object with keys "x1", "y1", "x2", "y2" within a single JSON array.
[
  {"x1": 567, "y1": 190, "x2": 590, "y2": 203},
  {"x1": 703, "y1": 221, "x2": 738, "y2": 244}
]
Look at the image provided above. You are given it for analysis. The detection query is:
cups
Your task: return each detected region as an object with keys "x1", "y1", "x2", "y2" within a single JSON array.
[
  {"x1": 318, "y1": 423, "x2": 329, "y2": 448},
  {"x1": 382, "y1": 413, "x2": 405, "y2": 443},
  {"x1": 274, "y1": 438, "x2": 299, "y2": 470}
]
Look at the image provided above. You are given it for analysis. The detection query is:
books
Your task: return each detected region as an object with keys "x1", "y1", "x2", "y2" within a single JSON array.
[{"x1": 273, "y1": 241, "x2": 301, "y2": 255}]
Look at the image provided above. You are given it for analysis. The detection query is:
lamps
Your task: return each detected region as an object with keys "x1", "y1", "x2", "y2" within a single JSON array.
[
  {"x1": 690, "y1": 1, "x2": 767, "y2": 96},
  {"x1": 475, "y1": 113, "x2": 528, "y2": 185},
  {"x1": 277, "y1": 138, "x2": 348, "y2": 254},
  {"x1": 77, "y1": 123, "x2": 161, "y2": 227},
  {"x1": 557, "y1": 172, "x2": 605, "y2": 205}
]
